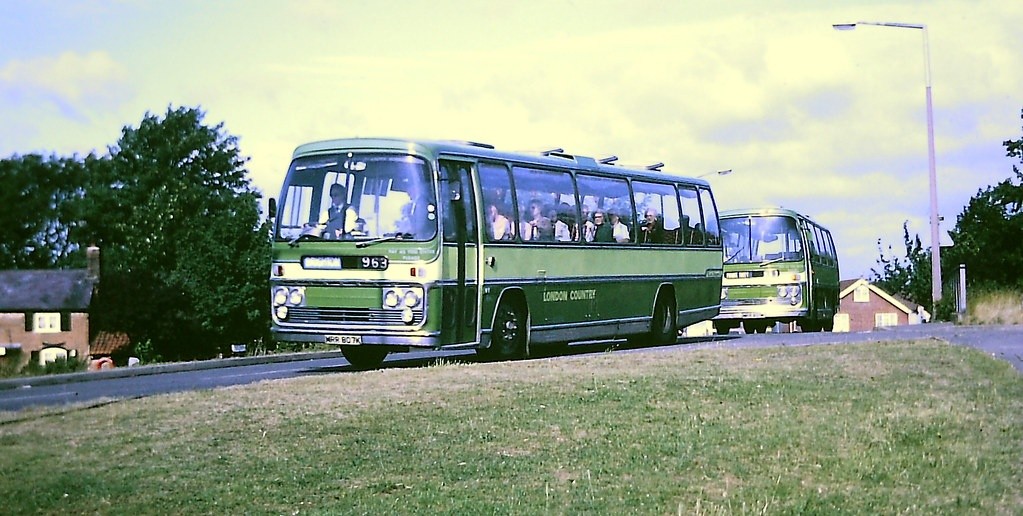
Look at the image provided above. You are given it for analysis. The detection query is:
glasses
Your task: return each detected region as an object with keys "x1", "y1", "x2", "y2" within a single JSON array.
[
  {"x1": 594, "y1": 215, "x2": 603, "y2": 219},
  {"x1": 330, "y1": 193, "x2": 340, "y2": 197}
]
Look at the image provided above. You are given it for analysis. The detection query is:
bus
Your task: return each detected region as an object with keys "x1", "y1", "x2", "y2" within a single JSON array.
[
  {"x1": 267, "y1": 137, "x2": 724, "y2": 362},
  {"x1": 704, "y1": 205, "x2": 841, "y2": 333}
]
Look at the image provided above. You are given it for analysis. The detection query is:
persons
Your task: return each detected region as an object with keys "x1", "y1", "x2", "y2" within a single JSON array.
[{"x1": 302, "y1": 181, "x2": 818, "y2": 259}]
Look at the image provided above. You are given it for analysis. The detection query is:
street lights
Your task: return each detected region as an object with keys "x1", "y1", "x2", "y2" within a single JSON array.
[{"x1": 832, "y1": 22, "x2": 942, "y2": 321}]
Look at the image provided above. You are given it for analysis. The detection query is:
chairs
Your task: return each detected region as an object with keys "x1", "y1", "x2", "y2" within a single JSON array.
[
  {"x1": 320, "y1": 208, "x2": 715, "y2": 247},
  {"x1": 729, "y1": 252, "x2": 837, "y2": 265}
]
